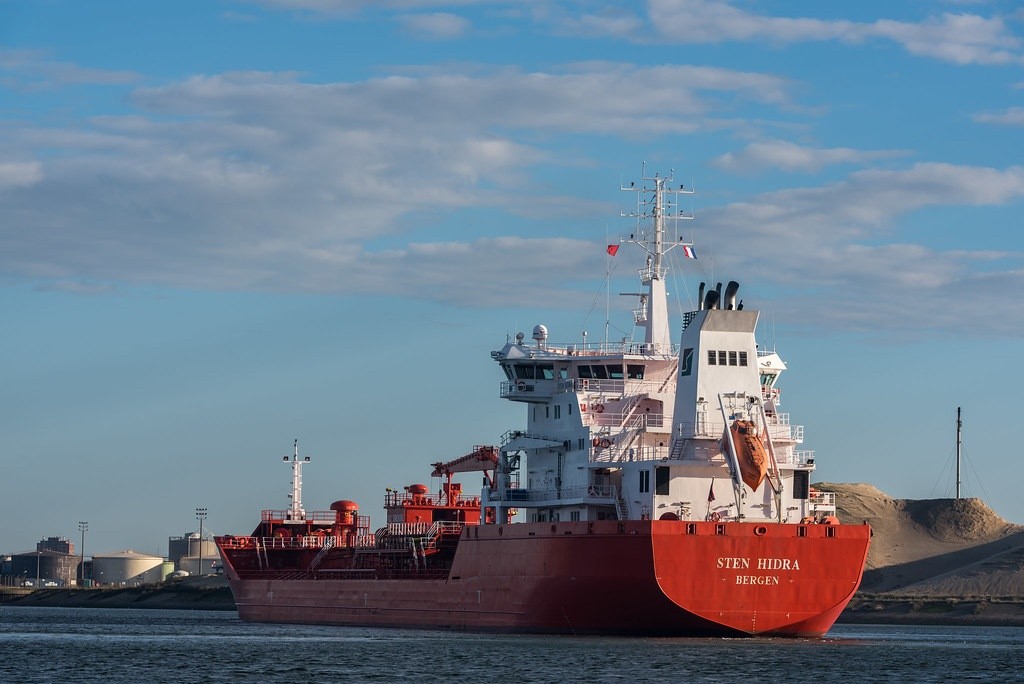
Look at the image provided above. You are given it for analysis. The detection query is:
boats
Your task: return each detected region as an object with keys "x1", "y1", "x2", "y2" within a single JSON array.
[{"x1": 213, "y1": 160, "x2": 873, "y2": 639}]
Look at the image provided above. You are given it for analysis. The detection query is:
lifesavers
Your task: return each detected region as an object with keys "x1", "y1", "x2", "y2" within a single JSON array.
[
  {"x1": 595, "y1": 405, "x2": 605, "y2": 413},
  {"x1": 712, "y1": 512, "x2": 721, "y2": 522},
  {"x1": 601, "y1": 439, "x2": 611, "y2": 448}
]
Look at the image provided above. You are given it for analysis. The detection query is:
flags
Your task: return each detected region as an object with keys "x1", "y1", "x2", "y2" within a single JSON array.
[
  {"x1": 684, "y1": 246, "x2": 697, "y2": 259},
  {"x1": 607, "y1": 245, "x2": 619, "y2": 256}
]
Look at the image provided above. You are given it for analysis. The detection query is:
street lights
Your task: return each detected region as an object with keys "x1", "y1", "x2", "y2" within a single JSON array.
[
  {"x1": 79, "y1": 522, "x2": 89, "y2": 580},
  {"x1": 196, "y1": 508, "x2": 207, "y2": 575}
]
